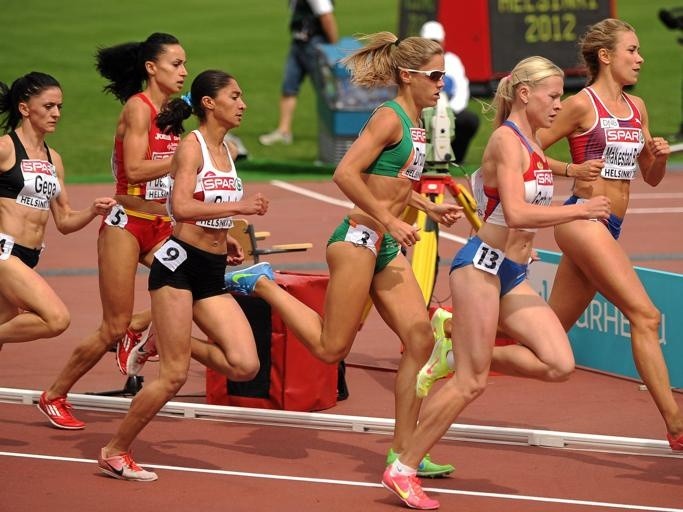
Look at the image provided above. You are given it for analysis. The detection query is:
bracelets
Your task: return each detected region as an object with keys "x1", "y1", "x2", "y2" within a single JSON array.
[{"x1": 564, "y1": 161, "x2": 572, "y2": 177}]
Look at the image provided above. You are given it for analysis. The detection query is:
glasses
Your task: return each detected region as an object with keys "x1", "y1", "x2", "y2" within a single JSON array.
[{"x1": 400, "y1": 67, "x2": 448, "y2": 84}]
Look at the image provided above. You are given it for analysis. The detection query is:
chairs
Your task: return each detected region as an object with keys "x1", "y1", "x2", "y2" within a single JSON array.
[{"x1": 229, "y1": 217, "x2": 314, "y2": 266}]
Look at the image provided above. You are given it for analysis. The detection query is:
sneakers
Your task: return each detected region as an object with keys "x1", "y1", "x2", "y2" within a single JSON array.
[
  {"x1": 662, "y1": 429, "x2": 682, "y2": 454},
  {"x1": 222, "y1": 260, "x2": 275, "y2": 296},
  {"x1": 415, "y1": 336, "x2": 453, "y2": 398},
  {"x1": 386, "y1": 446, "x2": 457, "y2": 478},
  {"x1": 124, "y1": 323, "x2": 162, "y2": 378},
  {"x1": 379, "y1": 465, "x2": 435, "y2": 508},
  {"x1": 259, "y1": 127, "x2": 293, "y2": 145},
  {"x1": 116, "y1": 326, "x2": 140, "y2": 373},
  {"x1": 33, "y1": 389, "x2": 82, "y2": 434},
  {"x1": 429, "y1": 305, "x2": 452, "y2": 336},
  {"x1": 94, "y1": 445, "x2": 160, "y2": 485}
]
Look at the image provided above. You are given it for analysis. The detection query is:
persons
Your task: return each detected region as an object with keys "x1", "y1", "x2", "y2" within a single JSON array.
[
  {"x1": 431, "y1": 19, "x2": 682, "y2": 453},
  {"x1": 258, "y1": 1, "x2": 338, "y2": 144},
  {"x1": 381, "y1": 56, "x2": 611, "y2": 511},
  {"x1": 224, "y1": 32, "x2": 465, "y2": 478},
  {"x1": 1, "y1": 72, "x2": 117, "y2": 346},
  {"x1": 420, "y1": 22, "x2": 480, "y2": 174},
  {"x1": 98, "y1": 69, "x2": 270, "y2": 481},
  {"x1": 36, "y1": 33, "x2": 188, "y2": 430}
]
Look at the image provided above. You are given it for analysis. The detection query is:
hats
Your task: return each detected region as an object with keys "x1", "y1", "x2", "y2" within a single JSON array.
[{"x1": 417, "y1": 18, "x2": 446, "y2": 42}]
